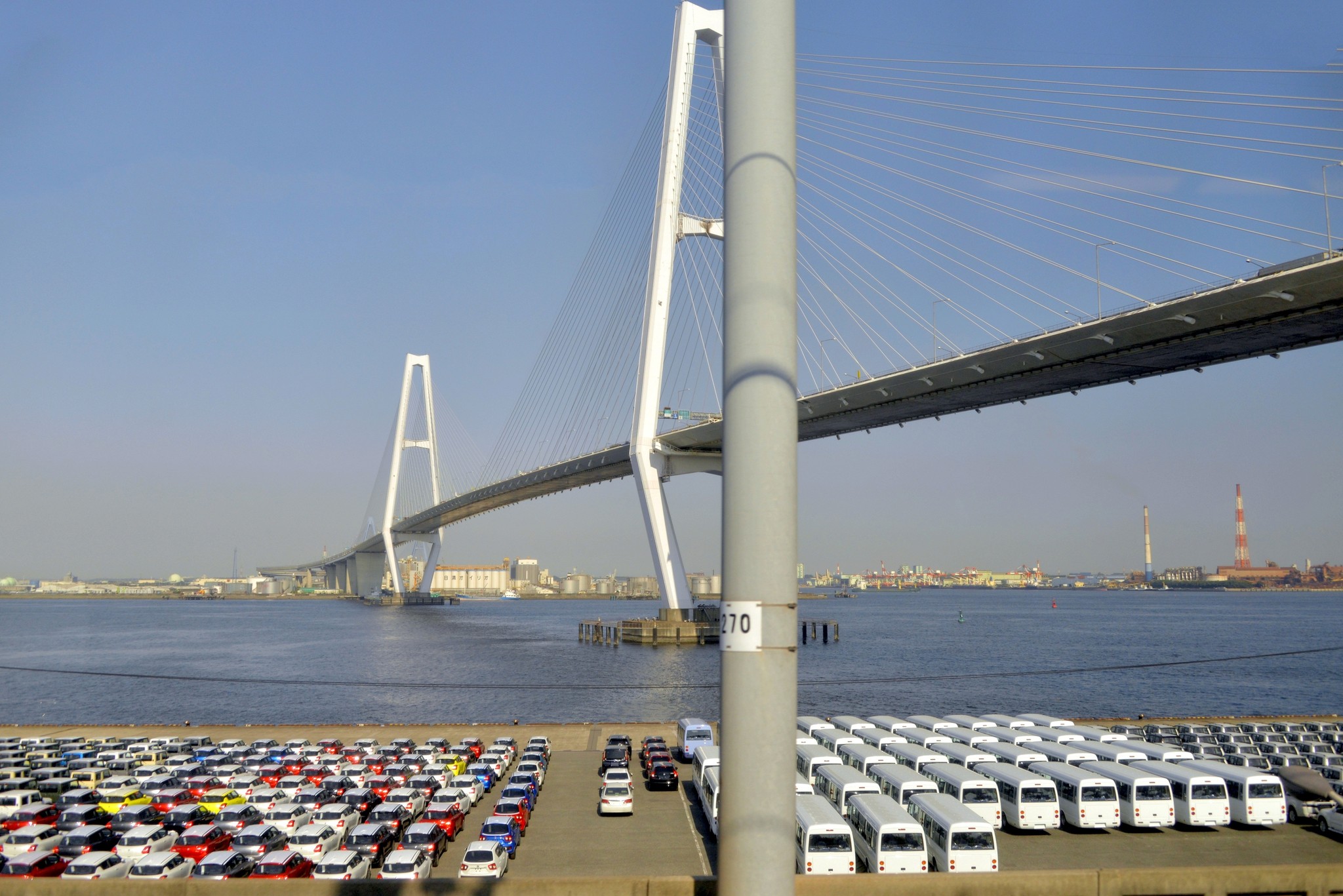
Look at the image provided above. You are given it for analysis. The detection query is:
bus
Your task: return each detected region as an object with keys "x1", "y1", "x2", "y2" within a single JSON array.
[{"x1": 677, "y1": 708, "x2": 1343, "y2": 877}]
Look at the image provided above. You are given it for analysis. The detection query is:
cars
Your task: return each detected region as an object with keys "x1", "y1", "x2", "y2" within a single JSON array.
[
  {"x1": 599, "y1": 732, "x2": 679, "y2": 814},
  {"x1": 0, "y1": 734, "x2": 553, "y2": 880}
]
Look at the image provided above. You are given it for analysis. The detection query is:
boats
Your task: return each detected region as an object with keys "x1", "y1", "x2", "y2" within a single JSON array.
[
  {"x1": 455, "y1": 593, "x2": 500, "y2": 599},
  {"x1": 364, "y1": 586, "x2": 384, "y2": 598},
  {"x1": 501, "y1": 588, "x2": 520, "y2": 600}
]
